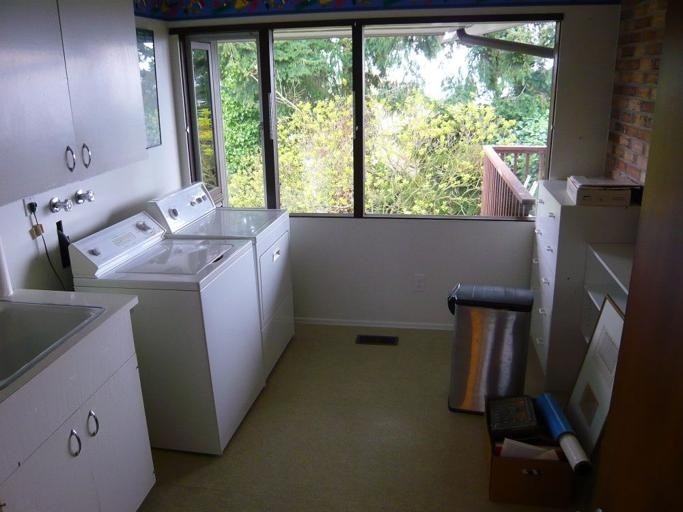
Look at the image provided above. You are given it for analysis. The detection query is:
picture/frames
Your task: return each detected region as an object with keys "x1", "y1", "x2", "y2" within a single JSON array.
[{"x1": 563, "y1": 293, "x2": 625, "y2": 464}]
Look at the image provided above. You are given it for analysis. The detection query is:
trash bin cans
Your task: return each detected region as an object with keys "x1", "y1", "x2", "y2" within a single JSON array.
[{"x1": 447, "y1": 280, "x2": 534, "y2": 416}]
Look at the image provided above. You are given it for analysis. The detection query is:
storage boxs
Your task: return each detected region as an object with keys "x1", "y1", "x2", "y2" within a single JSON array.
[{"x1": 489, "y1": 431, "x2": 574, "y2": 509}]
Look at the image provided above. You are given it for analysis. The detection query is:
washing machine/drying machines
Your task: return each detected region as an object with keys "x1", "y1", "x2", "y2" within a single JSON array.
[
  {"x1": 147, "y1": 182, "x2": 296, "y2": 381},
  {"x1": 67, "y1": 208, "x2": 266, "y2": 457}
]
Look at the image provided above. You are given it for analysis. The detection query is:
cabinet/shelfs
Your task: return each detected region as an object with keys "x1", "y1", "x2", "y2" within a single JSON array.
[
  {"x1": 525, "y1": 177, "x2": 641, "y2": 400},
  {"x1": 1, "y1": 310, "x2": 157, "y2": 512},
  {"x1": 577, "y1": 243, "x2": 635, "y2": 345},
  {"x1": 0, "y1": 0, "x2": 146, "y2": 208}
]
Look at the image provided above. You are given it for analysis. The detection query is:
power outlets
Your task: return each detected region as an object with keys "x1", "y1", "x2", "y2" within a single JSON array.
[{"x1": 23, "y1": 196, "x2": 38, "y2": 216}]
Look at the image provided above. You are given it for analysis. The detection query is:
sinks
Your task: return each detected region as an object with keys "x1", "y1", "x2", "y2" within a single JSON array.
[{"x1": 0, "y1": 299, "x2": 106, "y2": 391}]
